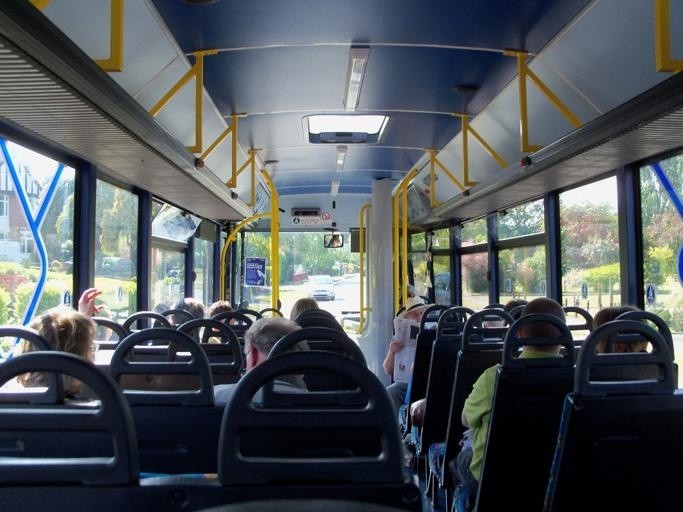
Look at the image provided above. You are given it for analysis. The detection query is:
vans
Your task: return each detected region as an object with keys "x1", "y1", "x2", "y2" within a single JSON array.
[{"x1": 307, "y1": 274, "x2": 335, "y2": 301}]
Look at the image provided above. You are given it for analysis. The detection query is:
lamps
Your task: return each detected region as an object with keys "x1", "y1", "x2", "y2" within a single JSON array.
[
  {"x1": 343, "y1": 46, "x2": 371, "y2": 111},
  {"x1": 335, "y1": 146, "x2": 348, "y2": 172},
  {"x1": 330, "y1": 180, "x2": 341, "y2": 197}
]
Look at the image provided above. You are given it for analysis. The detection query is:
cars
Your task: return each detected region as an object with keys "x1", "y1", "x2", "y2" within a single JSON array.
[{"x1": 424, "y1": 273, "x2": 452, "y2": 306}]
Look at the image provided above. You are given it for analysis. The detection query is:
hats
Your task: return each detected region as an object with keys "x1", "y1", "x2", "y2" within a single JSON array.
[{"x1": 399, "y1": 296, "x2": 435, "y2": 315}]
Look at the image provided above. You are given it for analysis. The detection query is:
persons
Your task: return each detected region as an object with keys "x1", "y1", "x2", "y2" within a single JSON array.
[
  {"x1": 330, "y1": 238, "x2": 341, "y2": 246},
  {"x1": 214, "y1": 317, "x2": 310, "y2": 404},
  {"x1": 383, "y1": 295, "x2": 529, "y2": 418},
  {"x1": 450, "y1": 298, "x2": 567, "y2": 489},
  {"x1": 14, "y1": 288, "x2": 104, "y2": 403},
  {"x1": 591, "y1": 305, "x2": 647, "y2": 354},
  {"x1": 290, "y1": 297, "x2": 319, "y2": 323},
  {"x1": 175, "y1": 298, "x2": 221, "y2": 344},
  {"x1": 209, "y1": 301, "x2": 235, "y2": 325}
]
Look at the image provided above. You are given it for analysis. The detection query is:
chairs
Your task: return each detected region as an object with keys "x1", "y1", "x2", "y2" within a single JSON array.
[
  {"x1": 387, "y1": 300, "x2": 682, "y2": 510},
  {"x1": 1, "y1": 302, "x2": 392, "y2": 510}
]
